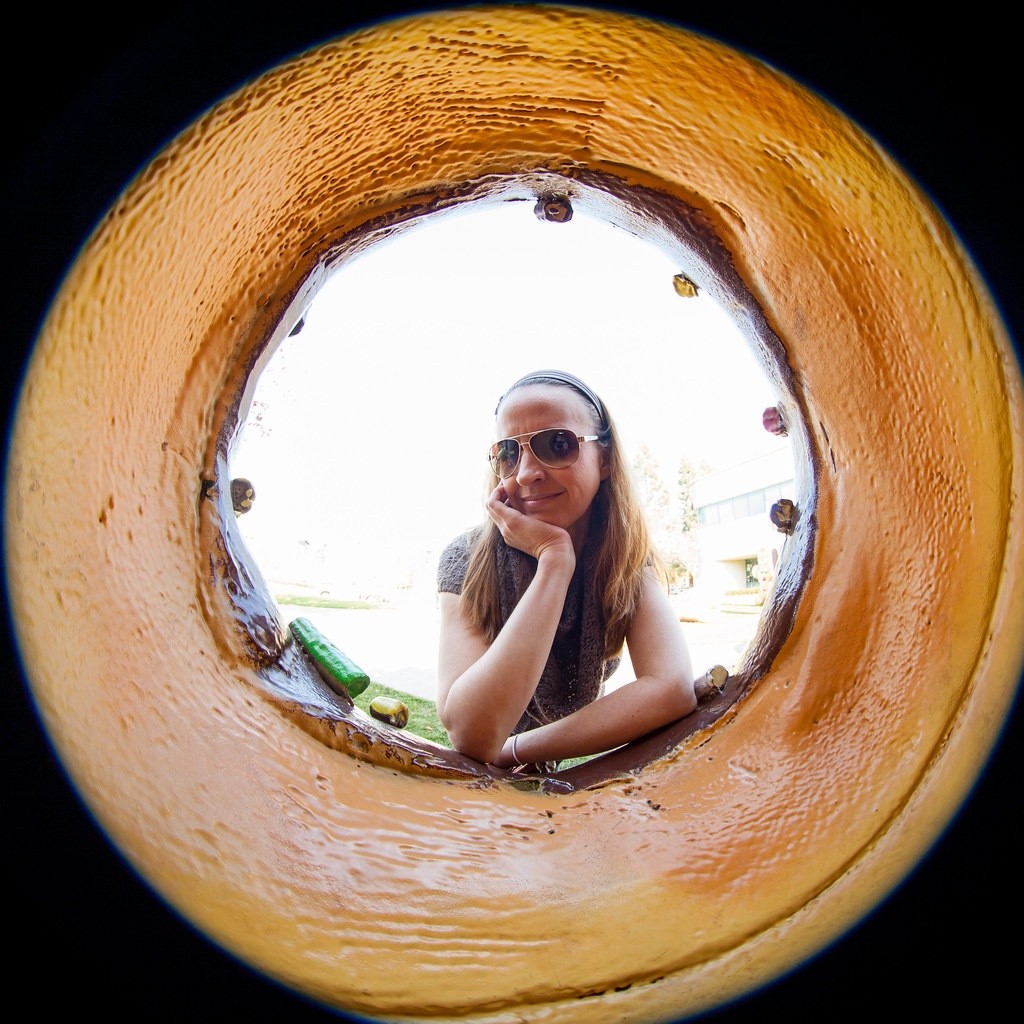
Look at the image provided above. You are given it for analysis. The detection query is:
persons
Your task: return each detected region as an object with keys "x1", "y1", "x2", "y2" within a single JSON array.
[{"x1": 434, "y1": 366, "x2": 698, "y2": 769}]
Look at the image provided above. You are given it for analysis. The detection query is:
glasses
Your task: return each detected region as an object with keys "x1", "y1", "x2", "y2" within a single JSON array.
[{"x1": 487, "y1": 428, "x2": 603, "y2": 479}]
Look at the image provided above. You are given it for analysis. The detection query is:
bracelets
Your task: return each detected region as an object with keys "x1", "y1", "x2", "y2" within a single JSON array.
[{"x1": 512, "y1": 734, "x2": 523, "y2": 765}]
